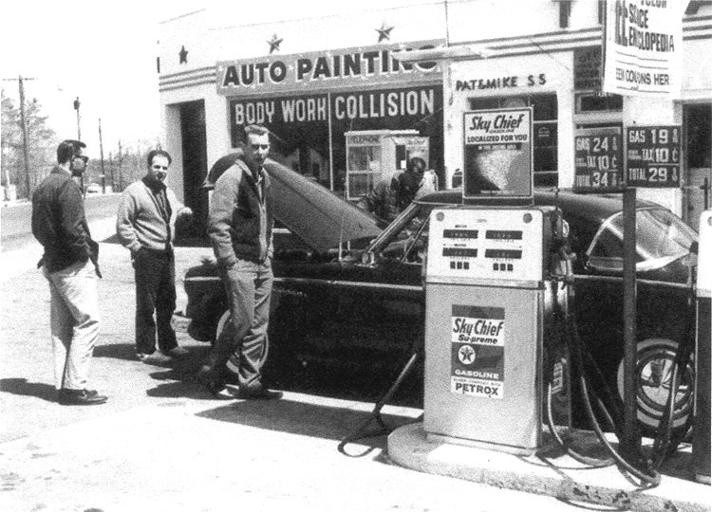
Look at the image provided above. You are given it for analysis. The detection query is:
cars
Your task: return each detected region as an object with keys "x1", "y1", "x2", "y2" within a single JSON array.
[{"x1": 171, "y1": 146, "x2": 699, "y2": 439}]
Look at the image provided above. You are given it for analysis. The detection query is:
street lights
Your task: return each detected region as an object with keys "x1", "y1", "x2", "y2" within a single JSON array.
[{"x1": 74, "y1": 98, "x2": 123, "y2": 194}]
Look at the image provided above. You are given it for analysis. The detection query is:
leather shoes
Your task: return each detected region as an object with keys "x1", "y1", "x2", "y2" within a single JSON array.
[
  {"x1": 198, "y1": 372, "x2": 236, "y2": 399},
  {"x1": 234, "y1": 386, "x2": 288, "y2": 401},
  {"x1": 57, "y1": 387, "x2": 108, "y2": 405}
]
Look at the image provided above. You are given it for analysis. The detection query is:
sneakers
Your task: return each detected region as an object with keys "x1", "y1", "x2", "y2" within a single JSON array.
[{"x1": 137, "y1": 347, "x2": 191, "y2": 363}]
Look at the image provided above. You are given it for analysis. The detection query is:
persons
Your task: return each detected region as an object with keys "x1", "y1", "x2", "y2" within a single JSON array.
[
  {"x1": 31, "y1": 140, "x2": 108, "y2": 406},
  {"x1": 198, "y1": 124, "x2": 282, "y2": 400},
  {"x1": 115, "y1": 150, "x2": 193, "y2": 367},
  {"x1": 356, "y1": 158, "x2": 426, "y2": 224}
]
